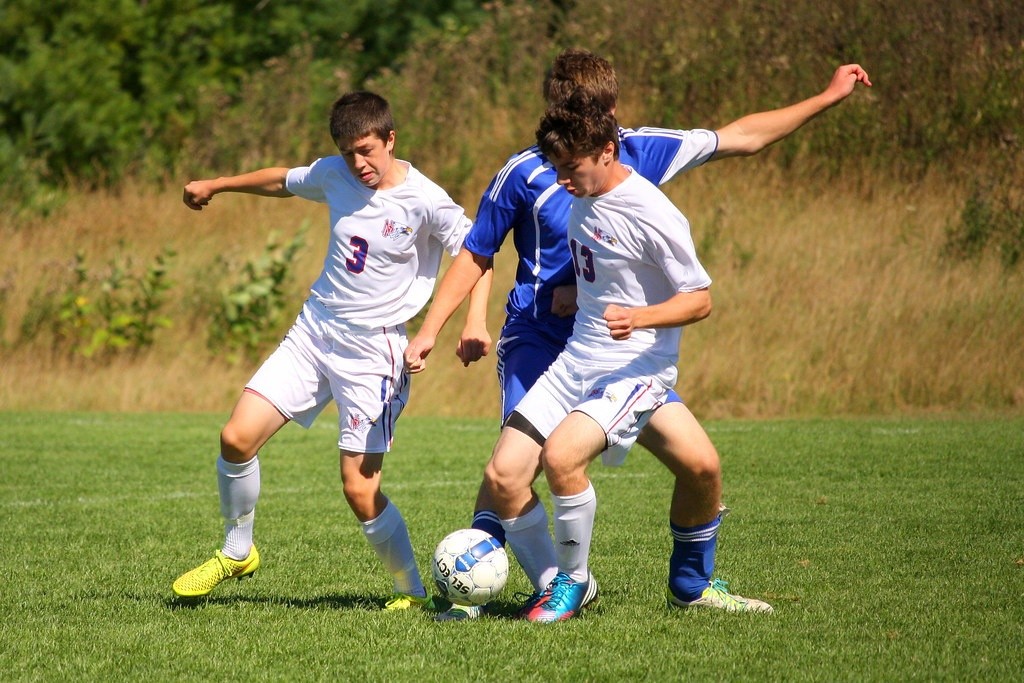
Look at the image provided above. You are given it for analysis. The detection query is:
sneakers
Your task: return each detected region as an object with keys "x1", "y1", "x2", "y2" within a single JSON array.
[
  {"x1": 433, "y1": 602, "x2": 485, "y2": 621},
  {"x1": 381, "y1": 585, "x2": 431, "y2": 612},
  {"x1": 514, "y1": 589, "x2": 557, "y2": 617},
  {"x1": 666, "y1": 577, "x2": 775, "y2": 616},
  {"x1": 172, "y1": 543, "x2": 261, "y2": 598},
  {"x1": 526, "y1": 570, "x2": 598, "y2": 624}
]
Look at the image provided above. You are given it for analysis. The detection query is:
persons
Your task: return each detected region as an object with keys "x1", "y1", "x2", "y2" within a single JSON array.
[{"x1": 172, "y1": 52, "x2": 873, "y2": 624}]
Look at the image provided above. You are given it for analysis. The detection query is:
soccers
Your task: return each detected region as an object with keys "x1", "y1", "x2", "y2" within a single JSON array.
[{"x1": 434, "y1": 527, "x2": 510, "y2": 605}]
